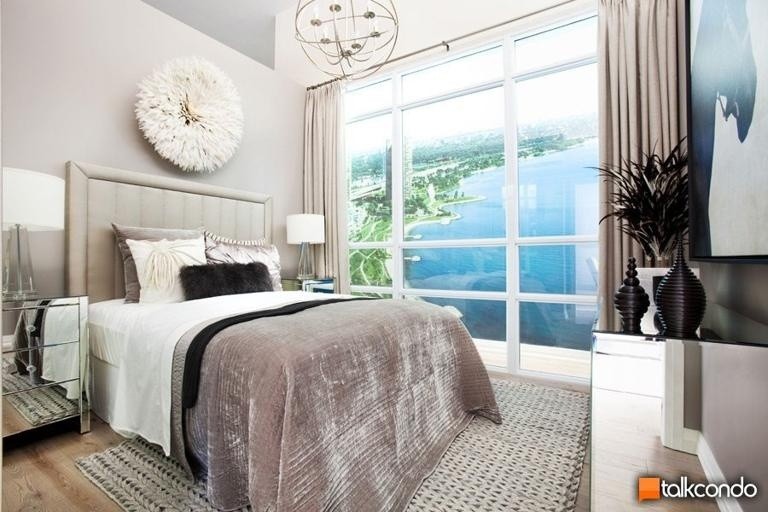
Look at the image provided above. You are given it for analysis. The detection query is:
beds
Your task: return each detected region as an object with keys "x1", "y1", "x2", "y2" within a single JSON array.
[{"x1": 65, "y1": 162, "x2": 502, "y2": 512}]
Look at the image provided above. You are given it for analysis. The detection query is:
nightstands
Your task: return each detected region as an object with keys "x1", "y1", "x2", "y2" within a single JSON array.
[{"x1": 0, "y1": 294, "x2": 92, "y2": 441}]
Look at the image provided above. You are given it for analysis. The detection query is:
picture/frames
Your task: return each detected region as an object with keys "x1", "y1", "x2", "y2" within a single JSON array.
[{"x1": 686, "y1": 1, "x2": 768, "y2": 265}]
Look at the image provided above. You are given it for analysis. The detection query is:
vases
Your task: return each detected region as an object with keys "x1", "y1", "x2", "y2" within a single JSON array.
[
  {"x1": 615, "y1": 257, "x2": 651, "y2": 335},
  {"x1": 654, "y1": 231, "x2": 706, "y2": 339}
]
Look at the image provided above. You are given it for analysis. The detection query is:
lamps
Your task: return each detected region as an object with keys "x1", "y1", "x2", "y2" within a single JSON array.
[
  {"x1": 285, "y1": 214, "x2": 325, "y2": 279},
  {"x1": 292, "y1": 1, "x2": 402, "y2": 81},
  {"x1": 0, "y1": 166, "x2": 64, "y2": 299}
]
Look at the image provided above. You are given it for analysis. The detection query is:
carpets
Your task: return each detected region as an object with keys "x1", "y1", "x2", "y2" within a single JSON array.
[
  {"x1": 73, "y1": 374, "x2": 591, "y2": 512},
  {"x1": 0, "y1": 356, "x2": 90, "y2": 428}
]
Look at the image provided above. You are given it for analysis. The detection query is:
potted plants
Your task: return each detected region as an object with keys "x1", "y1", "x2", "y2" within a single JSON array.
[{"x1": 584, "y1": 133, "x2": 703, "y2": 267}]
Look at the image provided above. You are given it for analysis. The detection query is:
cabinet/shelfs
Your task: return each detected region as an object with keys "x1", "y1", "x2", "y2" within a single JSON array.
[{"x1": 283, "y1": 279, "x2": 334, "y2": 293}]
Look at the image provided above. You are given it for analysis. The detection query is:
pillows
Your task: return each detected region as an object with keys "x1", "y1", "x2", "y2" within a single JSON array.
[
  {"x1": 110, "y1": 222, "x2": 206, "y2": 305},
  {"x1": 179, "y1": 260, "x2": 274, "y2": 300},
  {"x1": 125, "y1": 236, "x2": 207, "y2": 304},
  {"x1": 206, "y1": 230, "x2": 282, "y2": 292}
]
